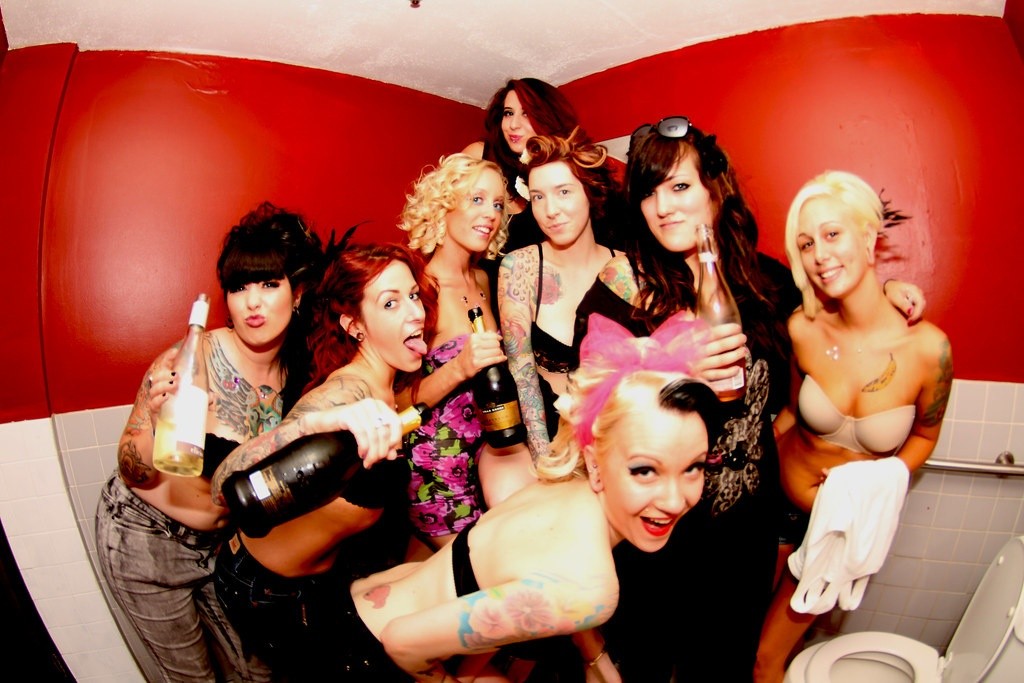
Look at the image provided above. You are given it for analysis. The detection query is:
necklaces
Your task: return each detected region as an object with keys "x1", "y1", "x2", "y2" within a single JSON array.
[
  {"x1": 453, "y1": 284, "x2": 486, "y2": 309},
  {"x1": 825, "y1": 344, "x2": 862, "y2": 361},
  {"x1": 257, "y1": 385, "x2": 280, "y2": 400}
]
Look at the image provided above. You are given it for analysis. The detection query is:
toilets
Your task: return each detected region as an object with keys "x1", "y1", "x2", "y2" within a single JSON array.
[{"x1": 781, "y1": 533, "x2": 1023, "y2": 683}]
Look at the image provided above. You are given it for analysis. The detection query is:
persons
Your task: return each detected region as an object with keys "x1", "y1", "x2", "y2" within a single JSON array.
[
  {"x1": 393, "y1": 150, "x2": 525, "y2": 561},
  {"x1": 251, "y1": 372, "x2": 727, "y2": 683},
  {"x1": 744, "y1": 168, "x2": 955, "y2": 680},
  {"x1": 567, "y1": 110, "x2": 805, "y2": 682},
  {"x1": 482, "y1": 76, "x2": 631, "y2": 334},
  {"x1": 196, "y1": 242, "x2": 442, "y2": 683},
  {"x1": 94, "y1": 198, "x2": 331, "y2": 683}
]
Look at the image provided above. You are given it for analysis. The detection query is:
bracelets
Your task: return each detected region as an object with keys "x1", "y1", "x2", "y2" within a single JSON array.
[
  {"x1": 585, "y1": 651, "x2": 607, "y2": 667},
  {"x1": 882, "y1": 277, "x2": 895, "y2": 296},
  {"x1": 410, "y1": 377, "x2": 421, "y2": 405}
]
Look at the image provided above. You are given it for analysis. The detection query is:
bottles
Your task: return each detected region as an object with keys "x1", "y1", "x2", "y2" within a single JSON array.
[
  {"x1": 222, "y1": 401, "x2": 434, "y2": 538},
  {"x1": 151, "y1": 292, "x2": 213, "y2": 476},
  {"x1": 691, "y1": 223, "x2": 745, "y2": 405},
  {"x1": 468, "y1": 307, "x2": 525, "y2": 447}
]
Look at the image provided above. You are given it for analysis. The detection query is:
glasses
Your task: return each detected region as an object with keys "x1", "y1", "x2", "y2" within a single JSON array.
[{"x1": 629, "y1": 117, "x2": 691, "y2": 152}]
